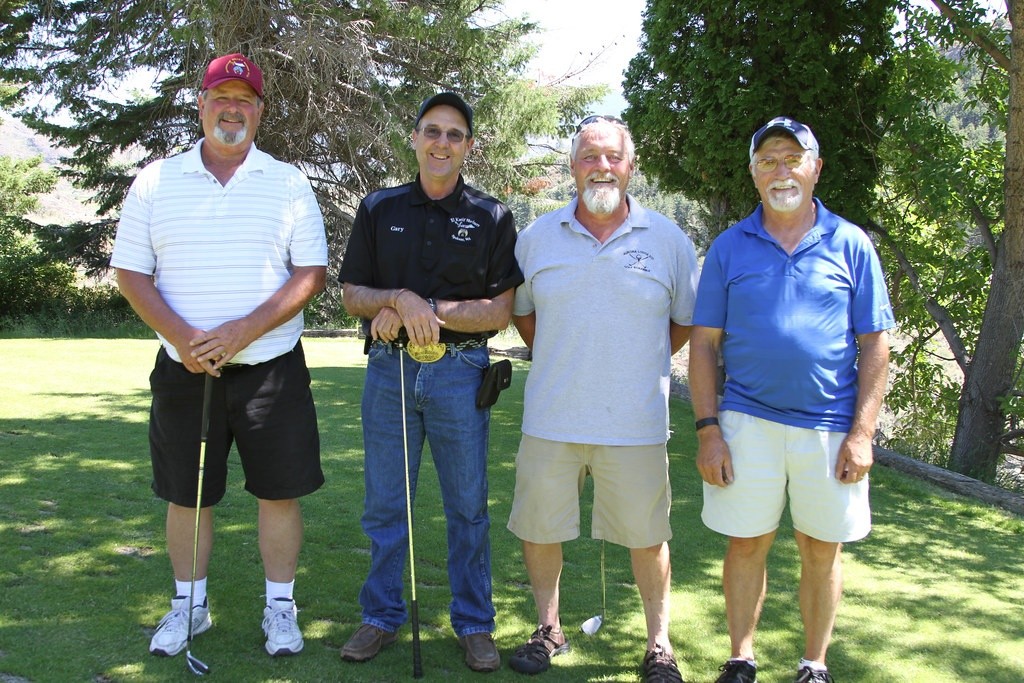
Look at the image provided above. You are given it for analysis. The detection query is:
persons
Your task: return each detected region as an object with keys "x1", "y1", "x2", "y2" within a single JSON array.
[
  {"x1": 109, "y1": 53, "x2": 328, "y2": 656},
  {"x1": 689, "y1": 116, "x2": 896, "y2": 683},
  {"x1": 509, "y1": 116, "x2": 699, "y2": 683},
  {"x1": 338, "y1": 94, "x2": 525, "y2": 673}
]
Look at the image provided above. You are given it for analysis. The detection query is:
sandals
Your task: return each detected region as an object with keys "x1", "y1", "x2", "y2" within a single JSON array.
[
  {"x1": 510, "y1": 617, "x2": 570, "y2": 673},
  {"x1": 643, "y1": 643, "x2": 682, "y2": 683}
]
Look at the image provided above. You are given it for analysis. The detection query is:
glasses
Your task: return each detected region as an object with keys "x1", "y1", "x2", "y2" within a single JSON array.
[
  {"x1": 416, "y1": 126, "x2": 467, "y2": 142},
  {"x1": 751, "y1": 155, "x2": 813, "y2": 172},
  {"x1": 572, "y1": 114, "x2": 633, "y2": 147}
]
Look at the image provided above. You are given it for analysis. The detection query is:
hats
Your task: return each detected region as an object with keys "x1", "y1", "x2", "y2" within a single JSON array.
[
  {"x1": 202, "y1": 52, "x2": 263, "y2": 101},
  {"x1": 415, "y1": 92, "x2": 473, "y2": 138},
  {"x1": 749, "y1": 117, "x2": 813, "y2": 160}
]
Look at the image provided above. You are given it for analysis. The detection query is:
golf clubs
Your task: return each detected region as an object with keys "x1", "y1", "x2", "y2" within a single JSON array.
[
  {"x1": 393, "y1": 334, "x2": 425, "y2": 679},
  {"x1": 581, "y1": 539, "x2": 606, "y2": 635},
  {"x1": 185, "y1": 358, "x2": 212, "y2": 676}
]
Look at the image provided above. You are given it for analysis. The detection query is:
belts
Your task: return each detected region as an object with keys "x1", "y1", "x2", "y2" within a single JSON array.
[{"x1": 371, "y1": 337, "x2": 487, "y2": 363}]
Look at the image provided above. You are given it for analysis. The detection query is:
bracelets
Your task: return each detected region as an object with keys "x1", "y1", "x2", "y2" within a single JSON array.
[
  {"x1": 425, "y1": 297, "x2": 436, "y2": 316},
  {"x1": 696, "y1": 417, "x2": 719, "y2": 432},
  {"x1": 394, "y1": 288, "x2": 408, "y2": 308}
]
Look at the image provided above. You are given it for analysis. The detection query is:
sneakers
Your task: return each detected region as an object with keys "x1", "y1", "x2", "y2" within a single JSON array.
[
  {"x1": 459, "y1": 632, "x2": 500, "y2": 671},
  {"x1": 149, "y1": 595, "x2": 213, "y2": 657},
  {"x1": 796, "y1": 666, "x2": 835, "y2": 683},
  {"x1": 341, "y1": 623, "x2": 397, "y2": 661},
  {"x1": 259, "y1": 593, "x2": 304, "y2": 656},
  {"x1": 714, "y1": 660, "x2": 757, "y2": 683}
]
